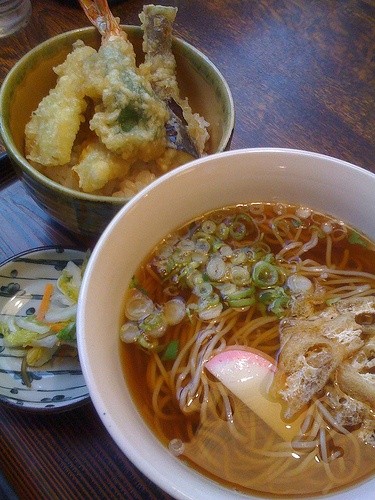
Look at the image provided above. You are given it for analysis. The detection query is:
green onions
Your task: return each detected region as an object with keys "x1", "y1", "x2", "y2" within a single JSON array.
[{"x1": 119, "y1": 205, "x2": 365, "y2": 348}]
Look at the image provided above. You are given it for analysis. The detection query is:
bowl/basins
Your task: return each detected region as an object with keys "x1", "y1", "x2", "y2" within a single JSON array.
[
  {"x1": 0, "y1": 24, "x2": 237, "y2": 237},
  {"x1": 76, "y1": 147, "x2": 375, "y2": 500}
]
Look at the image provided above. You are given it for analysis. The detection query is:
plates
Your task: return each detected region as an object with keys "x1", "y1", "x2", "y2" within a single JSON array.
[{"x1": 0, "y1": 247, "x2": 91, "y2": 411}]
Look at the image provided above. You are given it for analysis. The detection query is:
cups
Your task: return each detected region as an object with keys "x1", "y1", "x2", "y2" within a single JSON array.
[{"x1": 1, "y1": 0, "x2": 32, "y2": 37}]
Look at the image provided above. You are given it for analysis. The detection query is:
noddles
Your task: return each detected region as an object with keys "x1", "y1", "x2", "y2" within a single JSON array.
[{"x1": 123, "y1": 202, "x2": 374, "y2": 496}]
